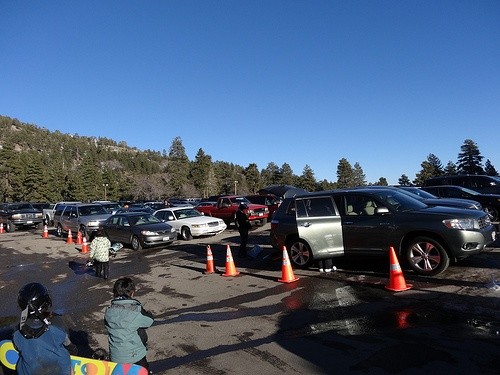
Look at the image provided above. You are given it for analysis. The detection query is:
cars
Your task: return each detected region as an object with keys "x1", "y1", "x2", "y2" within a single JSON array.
[{"x1": 99, "y1": 194, "x2": 284, "y2": 251}]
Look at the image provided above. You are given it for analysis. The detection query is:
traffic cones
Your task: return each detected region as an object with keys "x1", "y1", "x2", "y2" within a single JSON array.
[
  {"x1": 42, "y1": 224, "x2": 50, "y2": 239},
  {"x1": 221, "y1": 245, "x2": 241, "y2": 277},
  {"x1": 80, "y1": 235, "x2": 89, "y2": 255},
  {"x1": 0, "y1": 224, "x2": 5, "y2": 234},
  {"x1": 205, "y1": 244, "x2": 218, "y2": 274},
  {"x1": 278, "y1": 246, "x2": 300, "y2": 283},
  {"x1": 66, "y1": 228, "x2": 82, "y2": 246},
  {"x1": 384, "y1": 246, "x2": 413, "y2": 292}
]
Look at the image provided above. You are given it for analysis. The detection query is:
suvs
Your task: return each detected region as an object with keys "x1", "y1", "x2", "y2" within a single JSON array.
[
  {"x1": 256, "y1": 173, "x2": 500, "y2": 277},
  {"x1": 47, "y1": 200, "x2": 123, "y2": 241}
]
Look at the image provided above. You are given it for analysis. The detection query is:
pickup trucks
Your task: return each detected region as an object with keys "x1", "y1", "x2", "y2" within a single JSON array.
[{"x1": 0, "y1": 202, "x2": 44, "y2": 232}]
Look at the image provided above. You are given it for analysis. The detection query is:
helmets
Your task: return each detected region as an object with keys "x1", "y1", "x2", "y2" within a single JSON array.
[{"x1": 17, "y1": 282, "x2": 50, "y2": 318}]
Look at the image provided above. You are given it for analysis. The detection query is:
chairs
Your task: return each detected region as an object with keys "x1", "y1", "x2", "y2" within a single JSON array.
[
  {"x1": 347, "y1": 205, "x2": 357, "y2": 215},
  {"x1": 363, "y1": 201, "x2": 375, "y2": 216}
]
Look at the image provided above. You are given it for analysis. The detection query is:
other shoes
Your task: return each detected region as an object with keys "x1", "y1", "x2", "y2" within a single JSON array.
[{"x1": 103, "y1": 274, "x2": 108, "y2": 279}]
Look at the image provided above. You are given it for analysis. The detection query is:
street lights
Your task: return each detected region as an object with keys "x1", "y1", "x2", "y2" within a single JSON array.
[
  {"x1": 234, "y1": 180, "x2": 239, "y2": 196},
  {"x1": 102, "y1": 182, "x2": 109, "y2": 202}
]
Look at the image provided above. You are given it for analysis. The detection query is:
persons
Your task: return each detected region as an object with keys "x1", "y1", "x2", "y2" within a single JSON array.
[
  {"x1": 104, "y1": 277, "x2": 155, "y2": 375},
  {"x1": 237, "y1": 204, "x2": 252, "y2": 257},
  {"x1": 89, "y1": 229, "x2": 112, "y2": 280},
  {"x1": 311, "y1": 199, "x2": 337, "y2": 273},
  {"x1": 0, "y1": 282, "x2": 74, "y2": 375}
]
